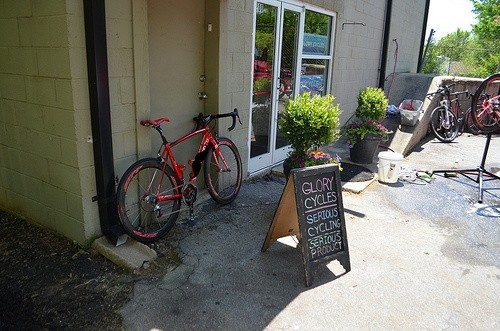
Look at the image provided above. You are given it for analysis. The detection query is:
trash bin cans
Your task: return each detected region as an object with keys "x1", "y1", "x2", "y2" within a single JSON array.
[{"x1": 382, "y1": 104, "x2": 401, "y2": 148}]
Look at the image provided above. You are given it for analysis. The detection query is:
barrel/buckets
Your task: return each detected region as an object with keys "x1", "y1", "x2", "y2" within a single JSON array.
[{"x1": 378, "y1": 151, "x2": 404, "y2": 183}]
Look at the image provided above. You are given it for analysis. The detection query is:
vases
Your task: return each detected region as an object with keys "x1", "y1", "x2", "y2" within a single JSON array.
[
  {"x1": 283, "y1": 158, "x2": 292, "y2": 183},
  {"x1": 350, "y1": 135, "x2": 383, "y2": 164}
]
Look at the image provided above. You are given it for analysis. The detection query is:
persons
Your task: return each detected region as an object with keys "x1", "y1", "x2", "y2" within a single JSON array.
[
  {"x1": 255, "y1": 43, "x2": 261, "y2": 59},
  {"x1": 259, "y1": 47, "x2": 268, "y2": 62}
]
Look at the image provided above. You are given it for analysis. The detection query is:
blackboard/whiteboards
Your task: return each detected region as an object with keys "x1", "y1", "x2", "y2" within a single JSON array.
[{"x1": 259, "y1": 161, "x2": 352, "y2": 289}]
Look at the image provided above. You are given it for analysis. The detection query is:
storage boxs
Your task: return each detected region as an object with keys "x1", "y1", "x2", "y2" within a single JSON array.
[{"x1": 399, "y1": 99, "x2": 424, "y2": 126}]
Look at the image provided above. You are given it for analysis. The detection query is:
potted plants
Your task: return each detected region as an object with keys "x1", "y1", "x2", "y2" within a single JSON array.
[
  {"x1": 276, "y1": 113, "x2": 289, "y2": 147},
  {"x1": 252, "y1": 78, "x2": 271, "y2": 145}
]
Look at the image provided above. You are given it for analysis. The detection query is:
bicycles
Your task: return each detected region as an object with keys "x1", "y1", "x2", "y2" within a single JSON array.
[
  {"x1": 425, "y1": 73, "x2": 500, "y2": 142},
  {"x1": 116, "y1": 107, "x2": 244, "y2": 243}
]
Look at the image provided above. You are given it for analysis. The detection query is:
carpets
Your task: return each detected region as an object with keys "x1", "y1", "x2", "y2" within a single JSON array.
[{"x1": 339, "y1": 162, "x2": 374, "y2": 182}]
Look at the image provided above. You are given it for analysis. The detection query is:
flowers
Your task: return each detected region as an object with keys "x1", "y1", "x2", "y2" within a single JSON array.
[
  {"x1": 278, "y1": 91, "x2": 343, "y2": 172},
  {"x1": 340, "y1": 86, "x2": 394, "y2": 148}
]
